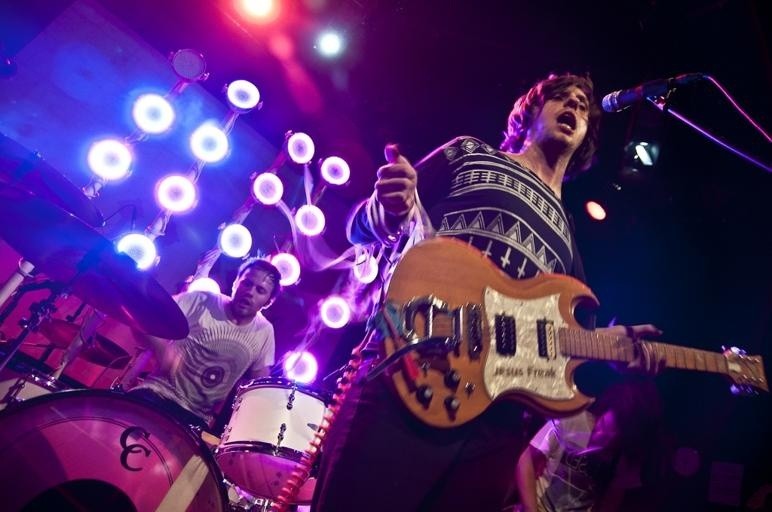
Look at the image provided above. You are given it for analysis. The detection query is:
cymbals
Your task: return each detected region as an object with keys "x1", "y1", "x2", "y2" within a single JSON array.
[
  {"x1": 38, "y1": 320, "x2": 132, "y2": 368},
  {"x1": 2, "y1": 183, "x2": 190, "y2": 339},
  {"x1": 1, "y1": 134, "x2": 105, "y2": 227}
]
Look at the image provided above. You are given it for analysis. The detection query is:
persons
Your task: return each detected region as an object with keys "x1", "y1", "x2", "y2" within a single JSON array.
[
  {"x1": 305, "y1": 67, "x2": 670, "y2": 511},
  {"x1": 123, "y1": 254, "x2": 282, "y2": 436},
  {"x1": 511, "y1": 377, "x2": 661, "y2": 512}
]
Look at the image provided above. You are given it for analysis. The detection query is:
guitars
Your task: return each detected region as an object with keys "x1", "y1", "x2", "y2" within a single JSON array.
[{"x1": 386, "y1": 237, "x2": 766, "y2": 428}]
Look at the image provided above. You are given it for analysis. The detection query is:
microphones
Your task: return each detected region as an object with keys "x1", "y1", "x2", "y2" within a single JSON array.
[{"x1": 600, "y1": 70, "x2": 704, "y2": 116}]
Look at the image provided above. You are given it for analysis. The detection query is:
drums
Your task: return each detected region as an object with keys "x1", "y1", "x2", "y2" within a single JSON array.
[
  {"x1": 1, "y1": 387, "x2": 231, "y2": 510},
  {"x1": 215, "y1": 377, "x2": 332, "y2": 505}
]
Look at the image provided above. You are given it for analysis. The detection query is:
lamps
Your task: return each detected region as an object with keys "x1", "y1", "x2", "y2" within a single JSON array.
[{"x1": 76, "y1": 48, "x2": 379, "y2": 388}]
